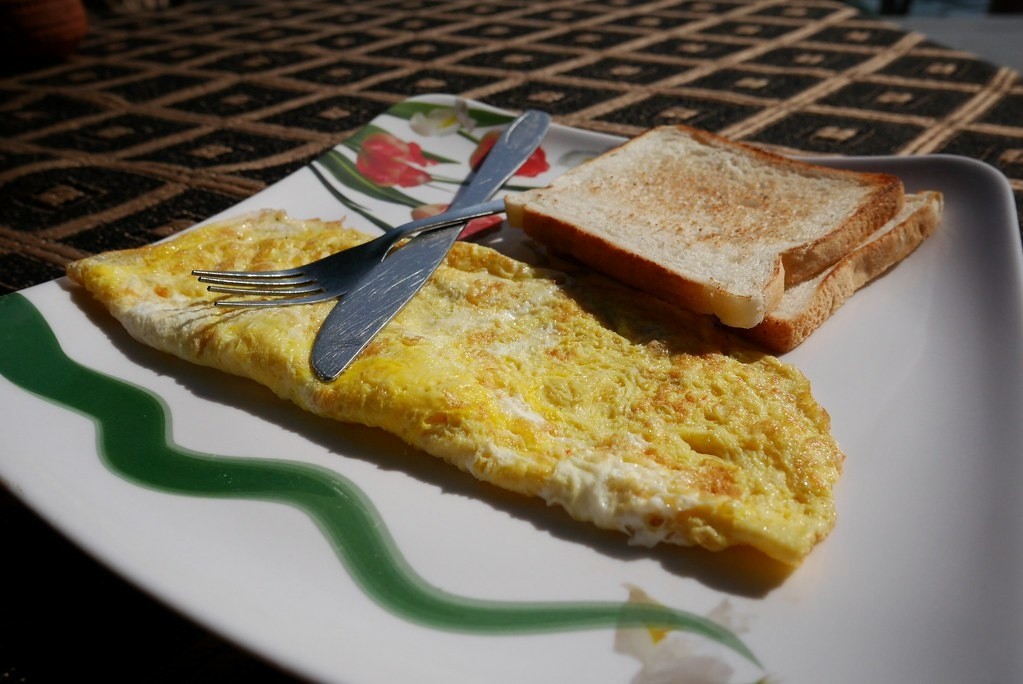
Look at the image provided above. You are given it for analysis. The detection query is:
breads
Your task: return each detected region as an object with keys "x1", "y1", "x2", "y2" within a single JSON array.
[{"x1": 506, "y1": 122, "x2": 938, "y2": 350}]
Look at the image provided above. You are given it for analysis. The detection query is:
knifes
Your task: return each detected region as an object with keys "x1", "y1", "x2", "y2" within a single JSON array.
[{"x1": 310, "y1": 109, "x2": 553, "y2": 382}]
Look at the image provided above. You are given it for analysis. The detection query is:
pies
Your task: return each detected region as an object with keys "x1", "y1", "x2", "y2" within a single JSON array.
[{"x1": 63, "y1": 208, "x2": 843, "y2": 564}]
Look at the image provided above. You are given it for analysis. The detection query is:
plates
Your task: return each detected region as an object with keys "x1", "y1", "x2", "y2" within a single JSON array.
[{"x1": 0, "y1": 94, "x2": 1023, "y2": 684}]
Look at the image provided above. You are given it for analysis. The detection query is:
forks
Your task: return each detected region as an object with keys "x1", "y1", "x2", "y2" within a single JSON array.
[{"x1": 192, "y1": 198, "x2": 506, "y2": 310}]
